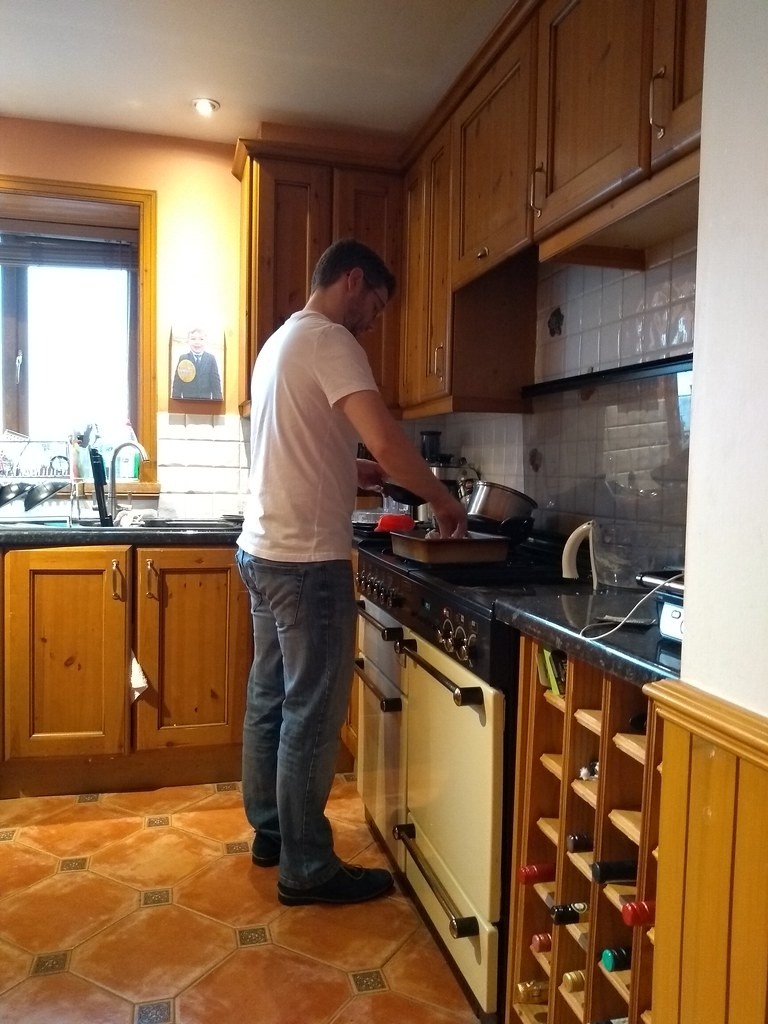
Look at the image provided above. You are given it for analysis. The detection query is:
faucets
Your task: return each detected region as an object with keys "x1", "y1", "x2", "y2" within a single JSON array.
[{"x1": 107, "y1": 439, "x2": 150, "y2": 523}]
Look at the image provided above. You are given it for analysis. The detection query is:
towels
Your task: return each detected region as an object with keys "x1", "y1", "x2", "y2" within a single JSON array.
[{"x1": 128, "y1": 648, "x2": 149, "y2": 707}]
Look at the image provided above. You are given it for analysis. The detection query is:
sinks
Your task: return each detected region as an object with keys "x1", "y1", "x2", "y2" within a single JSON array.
[
  {"x1": 139, "y1": 517, "x2": 240, "y2": 529},
  {"x1": 0, "y1": 519, "x2": 114, "y2": 528}
]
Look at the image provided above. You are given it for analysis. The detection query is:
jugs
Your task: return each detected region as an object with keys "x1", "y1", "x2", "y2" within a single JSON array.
[{"x1": 562, "y1": 518, "x2": 659, "y2": 588}]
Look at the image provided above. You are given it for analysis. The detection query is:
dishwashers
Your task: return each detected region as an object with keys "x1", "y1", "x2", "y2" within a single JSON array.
[{"x1": 357, "y1": 538, "x2": 408, "y2": 898}]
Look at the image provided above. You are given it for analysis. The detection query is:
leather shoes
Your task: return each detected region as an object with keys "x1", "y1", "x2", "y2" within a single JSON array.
[
  {"x1": 277, "y1": 862, "x2": 394, "y2": 907},
  {"x1": 251, "y1": 831, "x2": 282, "y2": 868}
]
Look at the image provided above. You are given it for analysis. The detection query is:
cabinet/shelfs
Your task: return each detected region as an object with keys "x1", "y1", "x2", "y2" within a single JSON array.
[
  {"x1": 0, "y1": 544, "x2": 262, "y2": 800},
  {"x1": 232, "y1": 0, "x2": 707, "y2": 421},
  {"x1": 504, "y1": 633, "x2": 678, "y2": 1024},
  {"x1": 349, "y1": 593, "x2": 503, "y2": 1024}
]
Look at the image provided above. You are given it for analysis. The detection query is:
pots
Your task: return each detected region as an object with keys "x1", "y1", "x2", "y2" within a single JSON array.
[{"x1": 459, "y1": 478, "x2": 538, "y2": 533}]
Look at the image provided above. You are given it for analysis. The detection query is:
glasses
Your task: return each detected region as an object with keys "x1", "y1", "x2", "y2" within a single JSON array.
[{"x1": 347, "y1": 270, "x2": 386, "y2": 321}]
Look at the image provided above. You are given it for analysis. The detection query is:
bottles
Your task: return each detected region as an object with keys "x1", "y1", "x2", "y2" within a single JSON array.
[
  {"x1": 517, "y1": 865, "x2": 555, "y2": 885},
  {"x1": 568, "y1": 834, "x2": 594, "y2": 853},
  {"x1": 622, "y1": 901, "x2": 655, "y2": 924},
  {"x1": 548, "y1": 902, "x2": 591, "y2": 925},
  {"x1": 563, "y1": 969, "x2": 586, "y2": 993},
  {"x1": 532, "y1": 933, "x2": 551, "y2": 953},
  {"x1": 602, "y1": 945, "x2": 631, "y2": 972},
  {"x1": 592, "y1": 859, "x2": 637, "y2": 883}
]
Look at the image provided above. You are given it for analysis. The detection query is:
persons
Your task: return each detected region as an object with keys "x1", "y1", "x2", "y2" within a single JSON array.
[
  {"x1": 172, "y1": 326, "x2": 222, "y2": 399},
  {"x1": 235, "y1": 237, "x2": 468, "y2": 906}
]
